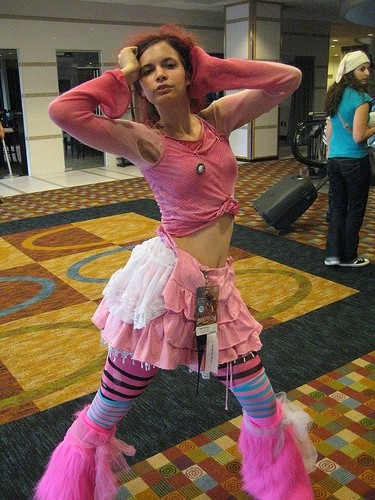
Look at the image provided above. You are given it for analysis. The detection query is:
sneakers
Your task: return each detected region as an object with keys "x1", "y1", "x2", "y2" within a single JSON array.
[
  {"x1": 339, "y1": 255, "x2": 370, "y2": 267},
  {"x1": 324, "y1": 255, "x2": 342, "y2": 266}
]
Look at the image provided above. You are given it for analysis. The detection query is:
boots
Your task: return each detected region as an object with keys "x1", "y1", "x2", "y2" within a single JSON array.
[
  {"x1": 236, "y1": 392, "x2": 318, "y2": 499},
  {"x1": 35, "y1": 405, "x2": 138, "y2": 500}
]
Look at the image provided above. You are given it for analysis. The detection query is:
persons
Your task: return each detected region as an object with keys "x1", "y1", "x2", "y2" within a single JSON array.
[
  {"x1": 32, "y1": 23, "x2": 320, "y2": 500},
  {"x1": 322, "y1": 50, "x2": 375, "y2": 267}
]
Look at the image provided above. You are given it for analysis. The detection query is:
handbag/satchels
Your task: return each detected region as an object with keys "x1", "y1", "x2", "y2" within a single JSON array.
[{"x1": 366, "y1": 111, "x2": 375, "y2": 147}]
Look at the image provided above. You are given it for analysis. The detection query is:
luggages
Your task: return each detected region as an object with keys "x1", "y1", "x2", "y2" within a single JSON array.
[{"x1": 253, "y1": 174, "x2": 329, "y2": 236}]
